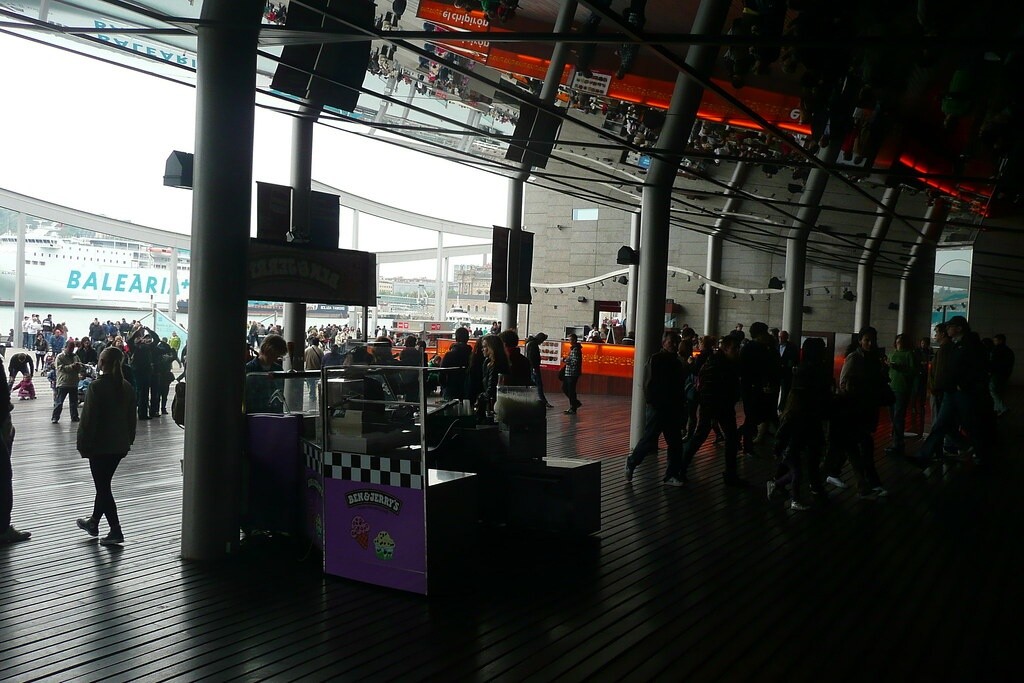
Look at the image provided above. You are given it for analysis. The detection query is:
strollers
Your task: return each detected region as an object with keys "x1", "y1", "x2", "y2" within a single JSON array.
[{"x1": 41, "y1": 352, "x2": 57, "y2": 377}]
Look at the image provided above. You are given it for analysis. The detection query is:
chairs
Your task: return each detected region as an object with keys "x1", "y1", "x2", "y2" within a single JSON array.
[{"x1": 395, "y1": 418, "x2": 461, "y2": 469}]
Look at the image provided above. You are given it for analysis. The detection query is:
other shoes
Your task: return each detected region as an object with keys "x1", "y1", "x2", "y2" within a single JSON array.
[
  {"x1": 625, "y1": 456, "x2": 633, "y2": 481},
  {"x1": 827, "y1": 476, "x2": 849, "y2": 488},
  {"x1": 52, "y1": 420, "x2": 58, "y2": 423},
  {"x1": 162, "y1": 409, "x2": 168, "y2": 414},
  {"x1": 140, "y1": 417, "x2": 152, "y2": 420},
  {"x1": 566, "y1": 410, "x2": 576, "y2": 415},
  {"x1": 152, "y1": 414, "x2": 161, "y2": 417},
  {"x1": 679, "y1": 470, "x2": 689, "y2": 481},
  {"x1": 662, "y1": 477, "x2": 685, "y2": 487},
  {"x1": 883, "y1": 448, "x2": 904, "y2": 454},
  {"x1": 576, "y1": 402, "x2": 582, "y2": 408},
  {"x1": 791, "y1": 500, "x2": 811, "y2": 510},
  {"x1": 0, "y1": 525, "x2": 31, "y2": 542},
  {"x1": 74, "y1": 416, "x2": 80, "y2": 422},
  {"x1": 725, "y1": 476, "x2": 748, "y2": 486},
  {"x1": 767, "y1": 481, "x2": 776, "y2": 501},
  {"x1": 859, "y1": 491, "x2": 885, "y2": 502}
]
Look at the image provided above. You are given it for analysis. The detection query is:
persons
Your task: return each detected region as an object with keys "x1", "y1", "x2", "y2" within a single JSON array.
[
  {"x1": 265, "y1": 3, "x2": 1023, "y2": 221},
  {"x1": 585, "y1": 323, "x2": 609, "y2": 343},
  {"x1": 562, "y1": 334, "x2": 582, "y2": 414},
  {"x1": 0, "y1": 355, "x2": 32, "y2": 544},
  {"x1": 885, "y1": 333, "x2": 916, "y2": 454},
  {"x1": 245, "y1": 323, "x2": 556, "y2": 428},
  {"x1": 6, "y1": 314, "x2": 187, "y2": 545},
  {"x1": 909, "y1": 316, "x2": 1015, "y2": 467},
  {"x1": 625, "y1": 322, "x2": 892, "y2": 510}
]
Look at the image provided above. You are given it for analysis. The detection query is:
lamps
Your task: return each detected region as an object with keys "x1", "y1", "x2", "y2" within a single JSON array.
[
  {"x1": 687, "y1": 276, "x2": 691, "y2": 282},
  {"x1": 670, "y1": 271, "x2": 677, "y2": 277},
  {"x1": 618, "y1": 275, "x2": 629, "y2": 286},
  {"x1": 163, "y1": 151, "x2": 193, "y2": 190},
  {"x1": 616, "y1": 245, "x2": 640, "y2": 267},
  {"x1": 578, "y1": 296, "x2": 586, "y2": 302},
  {"x1": 544, "y1": 288, "x2": 549, "y2": 294},
  {"x1": 766, "y1": 277, "x2": 967, "y2": 313},
  {"x1": 731, "y1": 292, "x2": 737, "y2": 299},
  {"x1": 697, "y1": 283, "x2": 707, "y2": 296},
  {"x1": 572, "y1": 287, "x2": 576, "y2": 294},
  {"x1": 715, "y1": 289, "x2": 720, "y2": 295},
  {"x1": 584, "y1": 284, "x2": 592, "y2": 290},
  {"x1": 532, "y1": 287, "x2": 537, "y2": 292},
  {"x1": 599, "y1": 280, "x2": 604, "y2": 288},
  {"x1": 558, "y1": 288, "x2": 564, "y2": 295},
  {"x1": 558, "y1": 146, "x2": 804, "y2": 224},
  {"x1": 611, "y1": 276, "x2": 617, "y2": 283},
  {"x1": 750, "y1": 295, "x2": 755, "y2": 301}
]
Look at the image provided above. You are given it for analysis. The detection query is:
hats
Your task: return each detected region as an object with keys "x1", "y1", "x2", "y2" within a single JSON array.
[{"x1": 944, "y1": 315, "x2": 967, "y2": 328}]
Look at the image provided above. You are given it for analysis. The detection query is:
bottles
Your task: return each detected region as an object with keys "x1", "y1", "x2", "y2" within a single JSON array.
[{"x1": 435, "y1": 399, "x2": 473, "y2": 417}]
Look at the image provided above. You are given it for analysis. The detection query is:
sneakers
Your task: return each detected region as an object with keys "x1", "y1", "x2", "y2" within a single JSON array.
[
  {"x1": 100, "y1": 524, "x2": 124, "y2": 544},
  {"x1": 78, "y1": 517, "x2": 99, "y2": 536}
]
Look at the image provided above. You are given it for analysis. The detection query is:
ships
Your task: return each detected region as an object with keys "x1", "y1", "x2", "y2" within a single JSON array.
[{"x1": 0, "y1": 218, "x2": 348, "y2": 312}]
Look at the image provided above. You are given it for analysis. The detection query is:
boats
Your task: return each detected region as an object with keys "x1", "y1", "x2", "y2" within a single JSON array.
[{"x1": 446, "y1": 296, "x2": 471, "y2": 327}]
